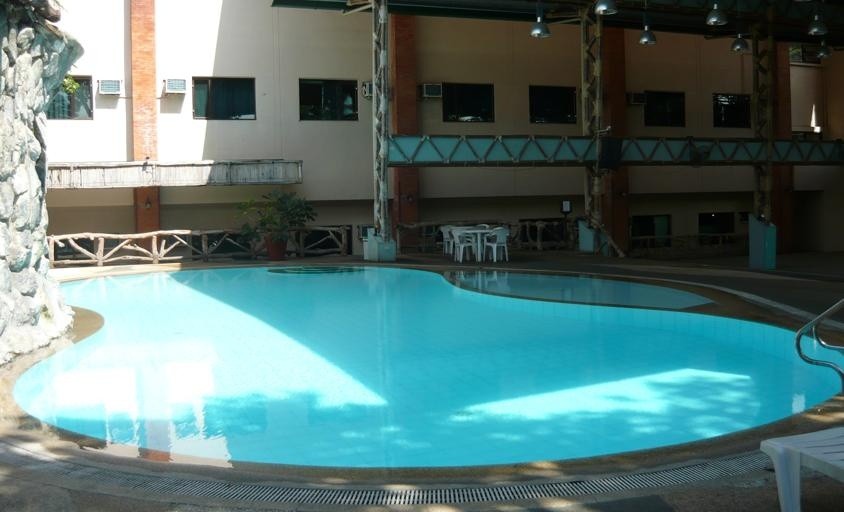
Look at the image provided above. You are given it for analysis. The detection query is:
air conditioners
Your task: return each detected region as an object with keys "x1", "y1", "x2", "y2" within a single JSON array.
[
  {"x1": 627, "y1": 89, "x2": 646, "y2": 107},
  {"x1": 421, "y1": 81, "x2": 444, "y2": 101},
  {"x1": 97, "y1": 77, "x2": 187, "y2": 96}
]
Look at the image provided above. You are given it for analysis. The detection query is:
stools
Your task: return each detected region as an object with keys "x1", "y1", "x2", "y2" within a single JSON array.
[{"x1": 755, "y1": 422, "x2": 844, "y2": 512}]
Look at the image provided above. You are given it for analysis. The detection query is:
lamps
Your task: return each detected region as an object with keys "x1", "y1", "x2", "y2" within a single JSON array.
[
  {"x1": 729, "y1": 0, "x2": 750, "y2": 52},
  {"x1": 595, "y1": 0, "x2": 618, "y2": 16},
  {"x1": 807, "y1": 1, "x2": 833, "y2": 60},
  {"x1": 637, "y1": 1, "x2": 659, "y2": 48},
  {"x1": 528, "y1": 0, "x2": 551, "y2": 40},
  {"x1": 705, "y1": 0, "x2": 726, "y2": 26}
]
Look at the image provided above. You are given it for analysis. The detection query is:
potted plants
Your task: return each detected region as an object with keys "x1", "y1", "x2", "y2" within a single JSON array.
[{"x1": 235, "y1": 186, "x2": 320, "y2": 262}]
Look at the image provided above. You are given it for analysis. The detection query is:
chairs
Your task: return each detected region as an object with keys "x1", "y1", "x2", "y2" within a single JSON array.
[{"x1": 434, "y1": 219, "x2": 513, "y2": 266}]
